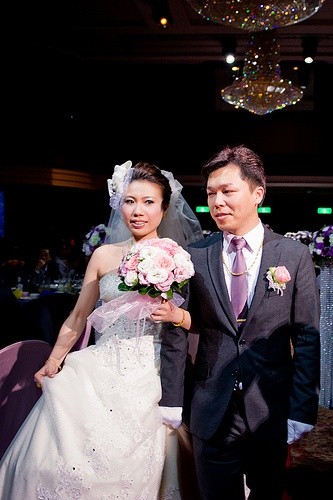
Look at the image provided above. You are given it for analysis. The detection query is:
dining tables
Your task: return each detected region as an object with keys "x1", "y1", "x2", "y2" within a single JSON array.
[{"x1": 0, "y1": 283, "x2": 96, "y2": 353}]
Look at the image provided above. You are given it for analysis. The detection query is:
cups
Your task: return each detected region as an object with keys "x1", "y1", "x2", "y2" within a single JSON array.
[
  {"x1": 53, "y1": 273, "x2": 84, "y2": 291},
  {"x1": 37, "y1": 275, "x2": 50, "y2": 291}
]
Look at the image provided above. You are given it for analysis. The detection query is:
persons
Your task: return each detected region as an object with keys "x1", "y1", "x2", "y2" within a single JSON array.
[
  {"x1": 0, "y1": 245, "x2": 89, "y2": 343},
  {"x1": 159, "y1": 147, "x2": 321, "y2": 500},
  {"x1": 0, "y1": 160, "x2": 203, "y2": 500}
]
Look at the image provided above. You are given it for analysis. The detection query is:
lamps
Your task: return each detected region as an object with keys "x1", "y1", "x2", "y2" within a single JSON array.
[{"x1": 188, "y1": 0, "x2": 326, "y2": 116}]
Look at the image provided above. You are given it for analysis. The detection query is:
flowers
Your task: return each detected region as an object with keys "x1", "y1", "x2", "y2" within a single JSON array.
[
  {"x1": 117, "y1": 238, "x2": 195, "y2": 301},
  {"x1": 82, "y1": 224, "x2": 114, "y2": 256},
  {"x1": 264, "y1": 266, "x2": 292, "y2": 296},
  {"x1": 282, "y1": 225, "x2": 333, "y2": 262},
  {"x1": 107, "y1": 160, "x2": 133, "y2": 197}
]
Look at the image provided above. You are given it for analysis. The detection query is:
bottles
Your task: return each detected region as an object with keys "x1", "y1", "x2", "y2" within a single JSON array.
[{"x1": 15, "y1": 277, "x2": 23, "y2": 292}]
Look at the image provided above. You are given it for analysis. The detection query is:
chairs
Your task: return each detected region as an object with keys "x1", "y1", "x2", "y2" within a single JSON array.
[{"x1": 0, "y1": 339, "x2": 53, "y2": 460}]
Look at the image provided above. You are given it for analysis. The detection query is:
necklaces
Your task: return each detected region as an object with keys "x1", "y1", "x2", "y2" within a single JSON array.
[{"x1": 222, "y1": 240, "x2": 263, "y2": 276}]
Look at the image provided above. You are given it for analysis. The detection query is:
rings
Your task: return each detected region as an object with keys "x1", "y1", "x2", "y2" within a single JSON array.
[{"x1": 37, "y1": 384, "x2": 41, "y2": 387}]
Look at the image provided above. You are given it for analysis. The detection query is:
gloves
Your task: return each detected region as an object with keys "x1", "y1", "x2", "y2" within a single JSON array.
[
  {"x1": 287, "y1": 419, "x2": 315, "y2": 444},
  {"x1": 159, "y1": 406, "x2": 183, "y2": 429}
]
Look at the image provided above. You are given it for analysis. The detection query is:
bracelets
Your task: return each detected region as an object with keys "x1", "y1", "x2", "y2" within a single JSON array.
[
  {"x1": 171, "y1": 307, "x2": 186, "y2": 327},
  {"x1": 45, "y1": 359, "x2": 62, "y2": 370}
]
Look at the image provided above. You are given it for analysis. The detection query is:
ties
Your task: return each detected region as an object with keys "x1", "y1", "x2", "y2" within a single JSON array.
[{"x1": 230, "y1": 238, "x2": 249, "y2": 331}]
